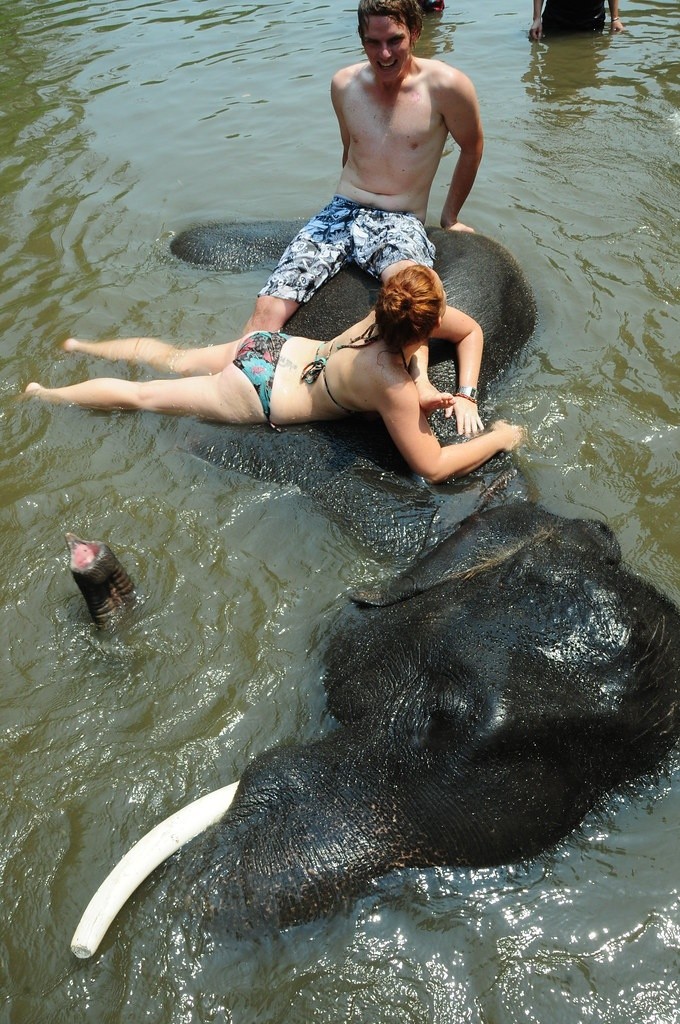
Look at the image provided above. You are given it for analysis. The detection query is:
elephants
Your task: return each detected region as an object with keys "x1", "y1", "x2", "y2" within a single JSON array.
[{"x1": 69, "y1": 218, "x2": 680, "y2": 963}]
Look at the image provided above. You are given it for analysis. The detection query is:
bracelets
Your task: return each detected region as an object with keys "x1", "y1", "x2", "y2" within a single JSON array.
[{"x1": 612, "y1": 17, "x2": 620, "y2": 21}]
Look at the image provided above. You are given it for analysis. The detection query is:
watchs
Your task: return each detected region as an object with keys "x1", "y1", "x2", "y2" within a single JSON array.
[{"x1": 455, "y1": 386, "x2": 479, "y2": 403}]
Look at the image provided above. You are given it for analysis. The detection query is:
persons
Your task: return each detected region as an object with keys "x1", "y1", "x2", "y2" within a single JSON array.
[
  {"x1": 240, "y1": 0, "x2": 484, "y2": 416},
  {"x1": 21, "y1": 264, "x2": 525, "y2": 483},
  {"x1": 529, "y1": 0, "x2": 623, "y2": 43}
]
